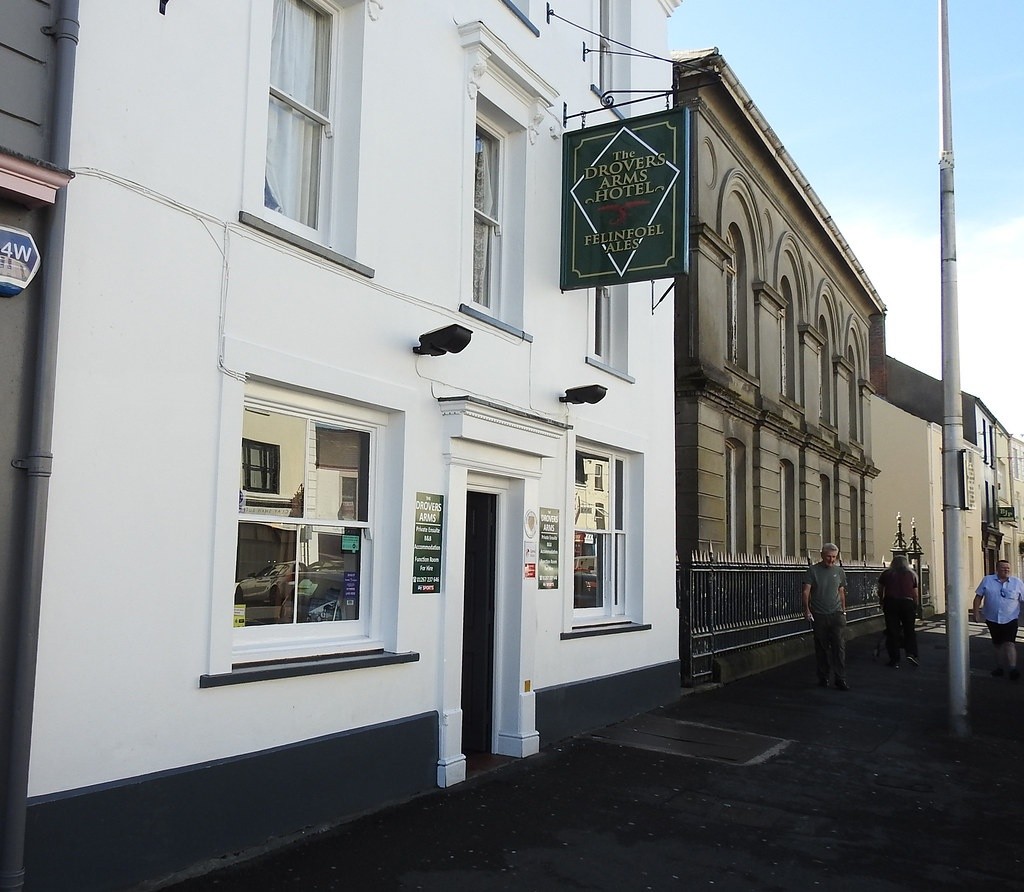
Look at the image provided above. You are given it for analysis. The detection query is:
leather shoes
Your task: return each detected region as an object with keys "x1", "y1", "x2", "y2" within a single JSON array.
[
  {"x1": 834, "y1": 679, "x2": 850, "y2": 689},
  {"x1": 820, "y1": 678, "x2": 831, "y2": 689}
]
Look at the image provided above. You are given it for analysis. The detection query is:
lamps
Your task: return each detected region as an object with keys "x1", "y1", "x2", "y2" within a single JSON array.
[
  {"x1": 558, "y1": 381, "x2": 608, "y2": 405},
  {"x1": 413, "y1": 323, "x2": 474, "y2": 354}
]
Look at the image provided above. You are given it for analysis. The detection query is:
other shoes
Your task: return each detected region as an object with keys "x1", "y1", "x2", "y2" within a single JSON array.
[
  {"x1": 1009, "y1": 669, "x2": 1020, "y2": 680},
  {"x1": 894, "y1": 661, "x2": 903, "y2": 671},
  {"x1": 906, "y1": 655, "x2": 919, "y2": 666},
  {"x1": 990, "y1": 669, "x2": 1004, "y2": 676}
]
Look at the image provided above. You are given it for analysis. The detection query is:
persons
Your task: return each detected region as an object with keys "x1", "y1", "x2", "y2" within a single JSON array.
[
  {"x1": 877, "y1": 555, "x2": 920, "y2": 670},
  {"x1": 974, "y1": 560, "x2": 1024, "y2": 680},
  {"x1": 803, "y1": 542, "x2": 850, "y2": 689}
]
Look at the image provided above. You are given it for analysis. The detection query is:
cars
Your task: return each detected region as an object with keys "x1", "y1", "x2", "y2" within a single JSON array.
[{"x1": 234, "y1": 559, "x2": 345, "y2": 624}]
